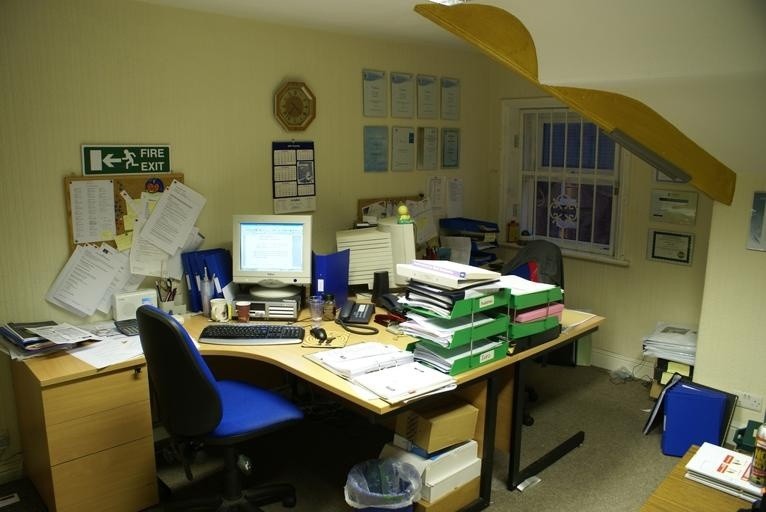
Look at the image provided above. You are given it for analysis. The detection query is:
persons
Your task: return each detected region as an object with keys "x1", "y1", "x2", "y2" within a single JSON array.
[{"x1": 121, "y1": 148, "x2": 139, "y2": 170}]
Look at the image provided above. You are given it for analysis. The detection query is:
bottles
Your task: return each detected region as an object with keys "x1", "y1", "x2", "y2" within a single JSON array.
[
  {"x1": 748, "y1": 422, "x2": 766, "y2": 489},
  {"x1": 200, "y1": 267, "x2": 212, "y2": 317}
]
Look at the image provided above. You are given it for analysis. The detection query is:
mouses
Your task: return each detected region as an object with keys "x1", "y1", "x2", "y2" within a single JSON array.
[{"x1": 310, "y1": 327, "x2": 327, "y2": 340}]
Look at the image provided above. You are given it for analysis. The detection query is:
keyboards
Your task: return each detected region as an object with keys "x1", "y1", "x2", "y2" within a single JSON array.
[{"x1": 198, "y1": 324, "x2": 305, "y2": 345}]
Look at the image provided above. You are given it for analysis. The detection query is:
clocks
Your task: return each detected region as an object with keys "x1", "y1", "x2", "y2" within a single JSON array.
[{"x1": 272, "y1": 81, "x2": 317, "y2": 131}]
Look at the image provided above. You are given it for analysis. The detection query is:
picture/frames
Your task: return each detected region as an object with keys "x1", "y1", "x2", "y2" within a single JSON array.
[{"x1": 745, "y1": 190, "x2": 766, "y2": 252}]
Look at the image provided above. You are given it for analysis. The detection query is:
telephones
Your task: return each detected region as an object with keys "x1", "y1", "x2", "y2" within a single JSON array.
[{"x1": 338, "y1": 300, "x2": 376, "y2": 325}]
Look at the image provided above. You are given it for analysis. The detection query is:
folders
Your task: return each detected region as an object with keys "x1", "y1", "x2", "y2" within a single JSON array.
[
  {"x1": 181, "y1": 248, "x2": 232, "y2": 312},
  {"x1": 396, "y1": 263, "x2": 501, "y2": 290},
  {"x1": 312, "y1": 252, "x2": 350, "y2": 309},
  {"x1": 643, "y1": 372, "x2": 729, "y2": 458}
]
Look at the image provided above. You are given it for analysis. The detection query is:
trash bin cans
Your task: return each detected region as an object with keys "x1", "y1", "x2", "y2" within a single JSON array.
[{"x1": 348, "y1": 459, "x2": 419, "y2": 512}]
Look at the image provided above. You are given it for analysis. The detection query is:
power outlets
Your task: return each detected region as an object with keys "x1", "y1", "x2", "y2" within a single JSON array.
[{"x1": 733, "y1": 389, "x2": 763, "y2": 412}]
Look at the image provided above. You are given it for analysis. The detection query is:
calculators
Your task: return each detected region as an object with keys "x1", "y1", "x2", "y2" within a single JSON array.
[{"x1": 115, "y1": 319, "x2": 140, "y2": 336}]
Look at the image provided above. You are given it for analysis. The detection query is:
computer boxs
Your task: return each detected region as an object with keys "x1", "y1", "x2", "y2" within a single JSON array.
[{"x1": 231, "y1": 294, "x2": 302, "y2": 320}]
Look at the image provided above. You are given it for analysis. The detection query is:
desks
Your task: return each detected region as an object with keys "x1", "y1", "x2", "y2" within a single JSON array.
[
  {"x1": 1, "y1": 268, "x2": 606, "y2": 512},
  {"x1": 637, "y1": 443, "x2": 766, "y2": 511}
]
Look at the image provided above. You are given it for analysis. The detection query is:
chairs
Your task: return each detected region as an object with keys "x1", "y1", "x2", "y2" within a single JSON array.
[
  {"x1": 502, "y1": 235, "x2": 564, "y2": 427},
  {"x1": 135, "y1": 302, "x2": 302, "y2": 512}
]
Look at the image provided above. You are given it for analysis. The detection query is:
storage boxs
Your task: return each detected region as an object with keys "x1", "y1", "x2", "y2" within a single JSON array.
[{"x1": 383, "y1": 396, "x2": 484, "y2": 512}]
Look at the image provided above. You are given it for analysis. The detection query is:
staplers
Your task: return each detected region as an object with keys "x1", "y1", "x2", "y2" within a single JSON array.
[{"x1": 375, "y1": 314, "x2": 404, "y2": 327}]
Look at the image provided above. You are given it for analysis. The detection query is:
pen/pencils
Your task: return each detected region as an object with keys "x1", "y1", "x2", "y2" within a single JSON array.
[{"x1": 155, "y1": 281, "x2": 177, "y2": 301}]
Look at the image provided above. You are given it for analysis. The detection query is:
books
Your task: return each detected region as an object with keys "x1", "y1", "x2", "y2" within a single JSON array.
[
  {"x1": 641, "y1": 321, "x2": 698, "y2": 368},
  {"x1": 0, "y1": 319, "x2": 78, "y2": 362},
  {"x1": 318, "y1": 340, "x2": 456, "y2": 405},
  {"x1": 393, "y1": 258, "x2": 558, "y2": 374},
  {"x1": 684, "y1": 440, "x2": 766, "y2": 503}
]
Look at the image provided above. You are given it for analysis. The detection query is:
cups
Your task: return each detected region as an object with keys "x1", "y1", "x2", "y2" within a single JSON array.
[
  {"x1": 159, "y1": 299, "x2": 173, "y2": 314},
  {"x1": 233, "y1": 301, "x2": 250, "y2": 322},
  {"x1": 208, "y1": 296, "x2": 227, "y2": 322},
  {"x1": 306, "y1": 295, "x2": 324, "y2": 321}
]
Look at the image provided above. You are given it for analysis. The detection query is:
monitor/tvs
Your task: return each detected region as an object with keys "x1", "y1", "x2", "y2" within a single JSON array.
[
  {"x1": 232, "y1": 213, "x2": 312, "y2": 299},
  {"x1": 336, "y1": 216, "x2": 417, "y2": 290}
]
